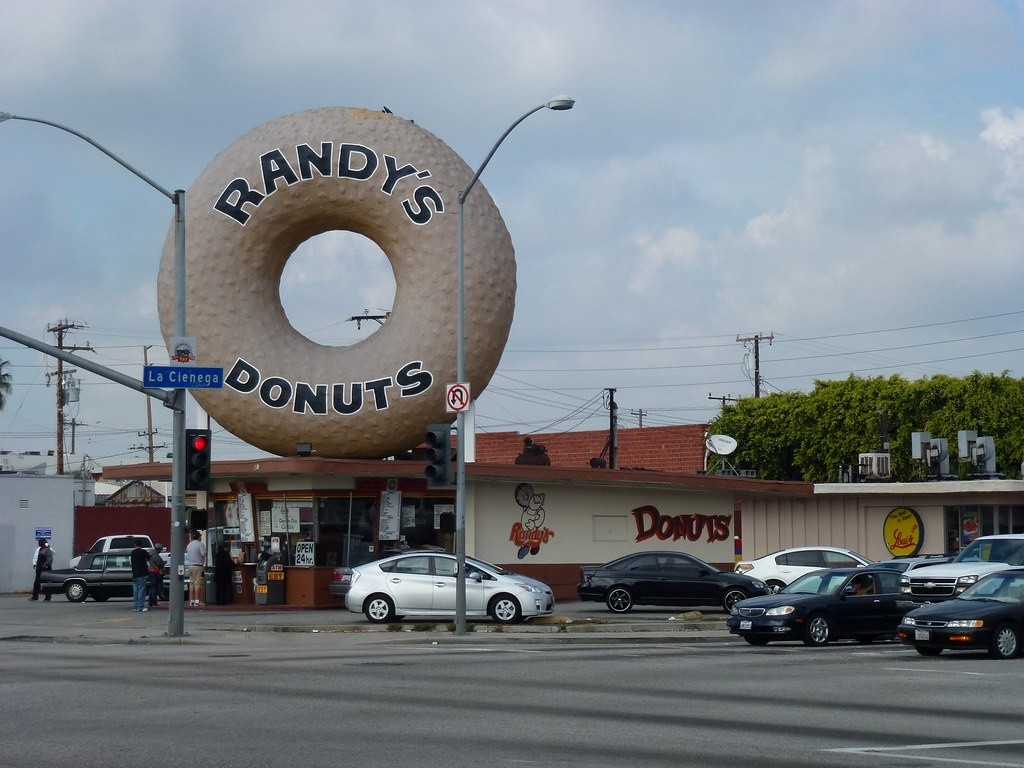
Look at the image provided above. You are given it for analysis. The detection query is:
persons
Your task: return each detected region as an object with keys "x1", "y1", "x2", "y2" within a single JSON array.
[
  {"x1": 130, "y1": 539, "x2": 151, "y2": 612},
  {"x1": 32, "y1": 540, "x2": 56, "y2": 569},
  {"x1": 213, "y1": 543, "x2": 238, "y2": 605},
  {"x1": 28, "y1": 537, "x2": 52, "y2": 602},
  {"x1": 852, "y1": 577, "x2": 867, "y2": 595},
  {"x1": 186, "y1": 531, "x2": 208, "y2": 607},
  {"x1": 149, "y1": 543, "x2": 168, "y2": 607}
]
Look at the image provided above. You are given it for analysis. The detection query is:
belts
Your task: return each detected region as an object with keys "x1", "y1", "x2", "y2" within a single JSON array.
[{"x1": 192, "y1": 564, "x2": 203, "y2": 566}]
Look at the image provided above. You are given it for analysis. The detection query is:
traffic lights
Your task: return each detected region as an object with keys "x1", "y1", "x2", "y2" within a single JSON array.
[
  {"x1": 423, "y1": 422, "x2": 453, "y2": 488},
  {"x1": 184, "y1": 427, "x2": 212, "y2": 489},
  {"x1": 187, "y1": 509, "x2": 208, "y2": 530}
]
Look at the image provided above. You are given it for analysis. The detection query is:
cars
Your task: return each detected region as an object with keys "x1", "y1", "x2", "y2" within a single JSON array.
[
  {"x1": 898, "y1": 569, "x2": 1024, "y2": 661},
  {"x1": 576, "y1": 549, "x2": 774, "y2": 616},
  {"x1": 39, "y1": 548, "x2": 169, "y2": 601},
  {"x1": 343, "y1": 551, "x2": 556, "y2": 623},
  {"x1": 728, "y1": 568, "x2": 909, "y2": 648},
  {"x1": 732, "y1": 546, "x2": 880, "y2": 595},
  {"x1": 327, "y1": 548, "x2": 415, "y2": 595}
]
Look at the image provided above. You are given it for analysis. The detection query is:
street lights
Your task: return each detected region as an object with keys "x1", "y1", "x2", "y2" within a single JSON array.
[
  {"x1": 455, "y1": 94, "x2": 579, "y2": 642},
  {"x1": 0, "y1": 112, "x2": 184, "y2": 638}
]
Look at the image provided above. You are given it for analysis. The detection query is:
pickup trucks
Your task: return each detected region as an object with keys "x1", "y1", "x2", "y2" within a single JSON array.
[{"x1": 68, "y1": 535, "x2": 188, "y2": 569}]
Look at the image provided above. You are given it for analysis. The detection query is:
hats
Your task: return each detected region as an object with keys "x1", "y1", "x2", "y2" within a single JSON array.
[
  {"x1": 155, "y1": 543, "x2": 163, "y2": 550},
  {"x1": 37, "y1": 537, "x2": 48, "y2": 543},
  {"x1": 192, "y1": 531, "x2": 201, "y2": 537}
]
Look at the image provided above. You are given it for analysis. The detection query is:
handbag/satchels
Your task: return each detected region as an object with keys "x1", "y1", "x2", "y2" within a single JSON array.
[{"x1": 159, "y1": 569, "x2": 166, "y2": 576}]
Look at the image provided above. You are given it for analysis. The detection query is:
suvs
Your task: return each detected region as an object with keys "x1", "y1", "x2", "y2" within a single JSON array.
[
  {"x1": 895, "y1": 532, "x2": 1024, "y2": 612},
  {"x1": 867, "y1": 552, "x2": 988, "y2": 574}
]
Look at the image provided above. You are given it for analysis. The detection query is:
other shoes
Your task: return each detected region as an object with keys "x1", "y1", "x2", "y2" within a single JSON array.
[
  {"x1": 133, "y1": 607, "x2": 139, "y2": 611},
  {"x1": 28, "y1": 598, "x2": 38, "y2": 601},
  {"x1": 137, "y1": 607, "x2": 150, "y2": 611},
  {"x1": 148, "y1": 602, "x2": 160, "y2": 607},
  {"x1": 43, "y1": 599, "x2": 50, "y2": 602},
  {"x1": 190, "y1": 602, "x2": 194, "y2": 606},
  {"x1": 194, "y1": 601, "x2": 206, "y2": 606}
]
